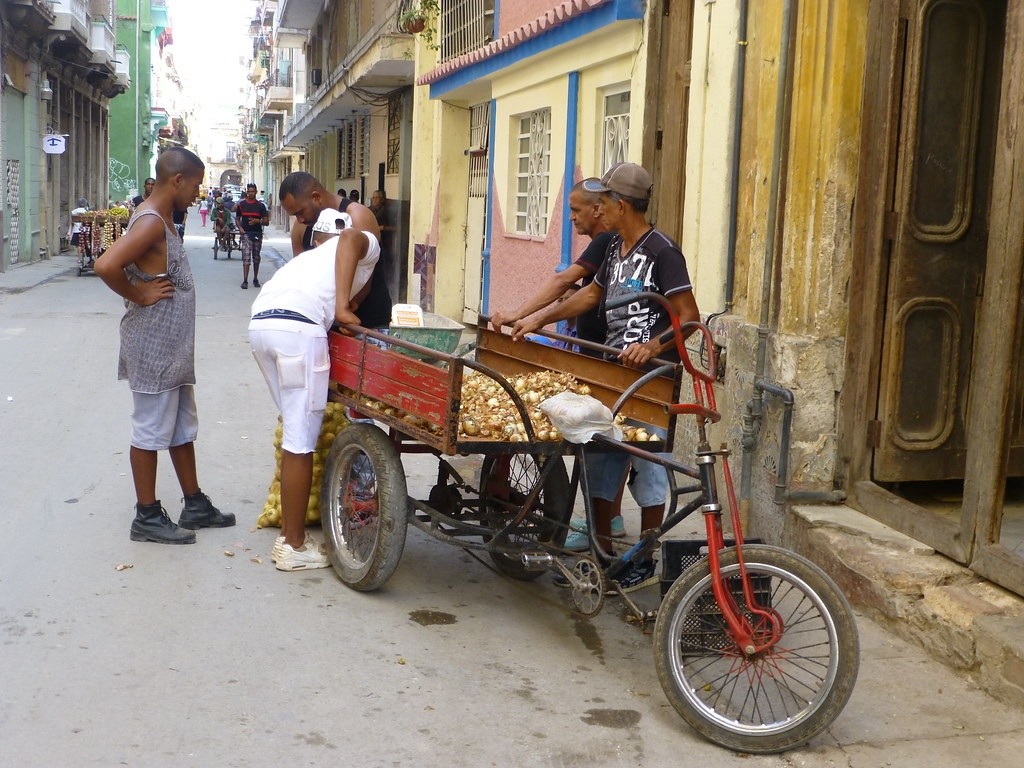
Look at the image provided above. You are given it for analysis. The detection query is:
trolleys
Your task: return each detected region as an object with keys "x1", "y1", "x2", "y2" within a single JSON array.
[{"x1": 72, "y1": 214, "x2": 129, "y2": 276}]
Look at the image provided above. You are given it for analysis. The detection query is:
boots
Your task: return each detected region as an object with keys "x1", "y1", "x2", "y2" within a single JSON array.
[
  {"x1": 178, "y1": 488, "x2": 236, "y2": 530},
  {"x1": 130, "y1": 500, "x2": 196, "y2": 544}
]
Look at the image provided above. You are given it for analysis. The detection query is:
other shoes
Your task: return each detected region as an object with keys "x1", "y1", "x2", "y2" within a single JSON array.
[
  {"x1": 241, "y1": 282, "x2": 248, "y2": 289},
  {"x1": 253, "y1": 280, "x2": 260, "y2": 287}
]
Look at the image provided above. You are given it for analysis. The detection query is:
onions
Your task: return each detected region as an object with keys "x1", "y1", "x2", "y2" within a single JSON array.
[
  {"x1": 79, "y1": 221, "x2": 121, "y2": 256},
  {"x1": 337, "y1": 383, "x2": 444, "y2": 436},
  {"x1": 460, "y1": 370, "x2": 662, "y2": 442},
  {"x1": 257, "y1": 401, "x2": 350, "y2": 529}
]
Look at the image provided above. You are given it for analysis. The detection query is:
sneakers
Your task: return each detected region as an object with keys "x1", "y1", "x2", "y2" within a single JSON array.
[
  {"x1": 569, "y1": 516, "x2": 626, "y2": 537},
  {"x1": 553, "y1": 551, "x2": 620, "y2": 588},
  {"x1": 562, "y1": 532, "x2": 590, "y2": 551},
  {"x1": 272, "y1": 530, "x2": 331, "y2": 570},
  {"x1": 603, "y1": 558, "x2": 659, "y2": 594}
]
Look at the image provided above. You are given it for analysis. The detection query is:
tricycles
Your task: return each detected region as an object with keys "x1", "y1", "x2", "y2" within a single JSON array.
[
  {"x1": 212, "y1": 223, "x2": 244, "y2": 261},
  {"x1": 320, "y1": 293, "x2": 862, "y2": 754}
]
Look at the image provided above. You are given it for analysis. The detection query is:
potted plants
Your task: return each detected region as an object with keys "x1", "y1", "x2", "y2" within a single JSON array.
[{"x1": 398, "y1": 0, "x2": 440, "y2": 58}]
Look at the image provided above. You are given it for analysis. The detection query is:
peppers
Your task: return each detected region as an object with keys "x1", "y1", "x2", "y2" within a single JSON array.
[{"x1": 108, "y1": 207, "x2": 130, "y2": 217}]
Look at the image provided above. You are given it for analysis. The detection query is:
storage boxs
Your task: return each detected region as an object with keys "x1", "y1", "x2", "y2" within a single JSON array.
[{"x1": 660, "y1": 538, "x2": 776, "y2": 656}]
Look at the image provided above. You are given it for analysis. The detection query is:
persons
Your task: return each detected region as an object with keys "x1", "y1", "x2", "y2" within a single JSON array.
[
  {"x1": 280, "y1": 172, "x2": 392, "y2": 527},
  {"x1": 552, "y1": 285, "x2": 582, "y2": 353},
  {"x1": 511, "y1": 161, "x2": 700, "y2": 599},
  {"x1": 249, "y1": 209, "x2": 382, "y2": 572},
  {"x1": 93, "y1": 145, "x2": 235, "y2": 544},
  {"x1": 69, "y1": 178, "x2": 271, "y2": 289},
  {"x1": 492, "y1": 178, "x2": 627, "y2": 550},
  {"x1": 337, "y1": 189, "x2": 389, "y2": 241}
]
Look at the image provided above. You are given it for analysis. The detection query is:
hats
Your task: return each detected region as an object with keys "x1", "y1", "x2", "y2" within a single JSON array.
[
  {"x1": 216, "y1": 197, "x2": 225, "y2": 204},
  {"x1": 310, "y1": 208, "x2": 353, "y2": 247},
  {"x1": 582, "y1": 163, "x2": 653, "y2": 200}
]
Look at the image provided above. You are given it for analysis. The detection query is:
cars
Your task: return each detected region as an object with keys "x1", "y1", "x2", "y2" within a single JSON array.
[{"x1": 224, "y1": 185, "x2": 244, "y2": 203}]
